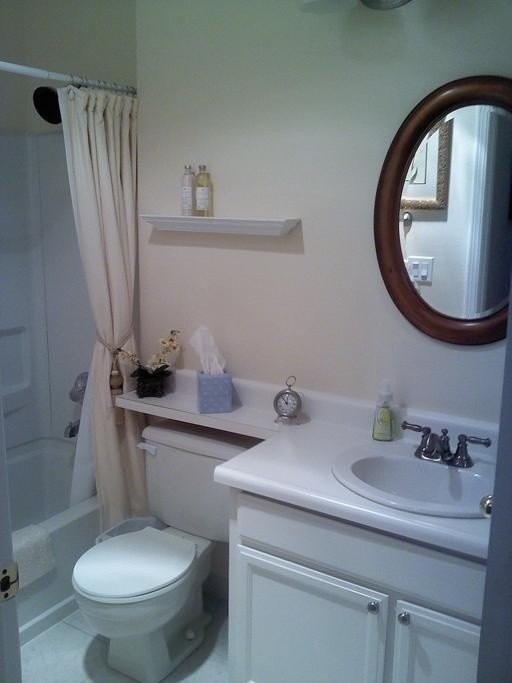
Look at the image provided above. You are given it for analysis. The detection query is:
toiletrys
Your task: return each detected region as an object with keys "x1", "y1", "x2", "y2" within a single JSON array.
[
  {"x1": 180, "y1": 164, "x2": 196, "y2": 216},
  {"x1": 195, "y1": 164, "x2": 212, "y2": 217}
]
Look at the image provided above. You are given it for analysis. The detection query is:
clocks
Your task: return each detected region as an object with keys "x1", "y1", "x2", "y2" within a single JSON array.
[{"x1": 273, "y1": 375, "x2": 303, "y2": 424}]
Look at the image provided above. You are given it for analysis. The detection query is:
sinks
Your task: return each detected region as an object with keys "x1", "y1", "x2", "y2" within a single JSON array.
[{"x1": 331, "y1": 438, "x2": 495, "y2": 519}]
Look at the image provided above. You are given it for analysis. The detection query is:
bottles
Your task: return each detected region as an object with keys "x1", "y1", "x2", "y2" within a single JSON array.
[{"x1": 178, "y1": 163, "x2": 214, "y2": 218}]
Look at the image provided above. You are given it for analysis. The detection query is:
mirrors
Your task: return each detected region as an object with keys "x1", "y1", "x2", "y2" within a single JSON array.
[{"x1": 373, "y1": 75, "x2": 512, "y2": 345}]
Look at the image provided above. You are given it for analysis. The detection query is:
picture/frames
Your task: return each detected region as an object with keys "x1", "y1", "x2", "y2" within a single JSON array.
[{"x1": 399, "y1": 111, "x2": 454, "y2": 209}]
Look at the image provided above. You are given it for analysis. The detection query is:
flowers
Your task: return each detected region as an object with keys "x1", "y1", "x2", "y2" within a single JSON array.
[{"x1": 113, "y1": 328, "x2": 180, "y2": 370}]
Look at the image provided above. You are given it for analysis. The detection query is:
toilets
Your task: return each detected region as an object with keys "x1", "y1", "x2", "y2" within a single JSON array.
[{"x1": 73, "y1": 424, "x2": 249, "y2": 681}]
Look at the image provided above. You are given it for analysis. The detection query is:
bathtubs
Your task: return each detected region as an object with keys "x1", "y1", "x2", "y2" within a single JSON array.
[{"x1": 5, "y1": 435, "x2": 100, "y2": 647}]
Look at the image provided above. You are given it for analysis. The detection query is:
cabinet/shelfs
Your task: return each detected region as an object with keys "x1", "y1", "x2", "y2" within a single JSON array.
[{"x1": 226, "y1": 542, "x2": 487, "y2": 683}]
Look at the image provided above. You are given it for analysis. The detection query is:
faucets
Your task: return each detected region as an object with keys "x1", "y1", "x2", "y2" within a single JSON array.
[
  {"x1": 63, "y1": 419, "x2": 81, "y2": 439},
  {"x1": 423, "y1": 424, "x2": 453, "y2": 462}
]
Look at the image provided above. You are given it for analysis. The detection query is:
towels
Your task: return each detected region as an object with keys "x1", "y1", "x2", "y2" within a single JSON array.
[{"x1": 12, "y1": 521, "x2": 57, "y2": 589}]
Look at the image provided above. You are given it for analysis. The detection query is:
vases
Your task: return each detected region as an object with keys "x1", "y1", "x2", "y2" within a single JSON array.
[{"x1": 129, "y1": 366, "x2": 171, "y2": 397}]
